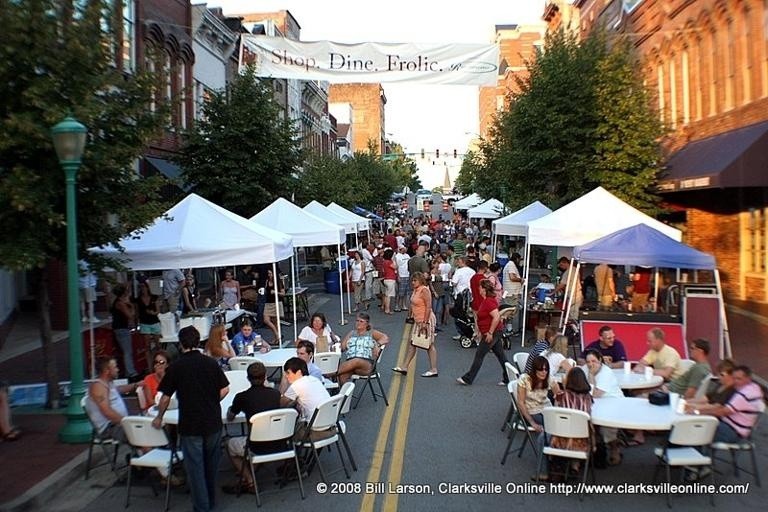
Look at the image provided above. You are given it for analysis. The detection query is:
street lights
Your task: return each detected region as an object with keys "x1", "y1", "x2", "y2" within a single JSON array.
[
  {"x1": 40, "y1": 111, "x2": 104, "y2": 450},
  {"x1": 500, "y1": 179, "x2": 509, "y2": 251}
]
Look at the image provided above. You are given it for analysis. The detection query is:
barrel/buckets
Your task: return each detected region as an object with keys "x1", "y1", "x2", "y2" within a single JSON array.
[
  {"x1": 324, "y1": 270, "x2": 339, "y2": 294},
  {"x1": 537, "y1": 283, "x2": 555, "y2": 302},
  {"x1": 497, "y1": 254, "x2": 508, "y2": 265}
]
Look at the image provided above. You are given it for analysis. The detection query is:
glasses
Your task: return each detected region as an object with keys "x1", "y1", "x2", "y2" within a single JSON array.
[
  {"x1": 538, "y1": 368, "x2": 549, "y2": 371},
  {"x1": 155, "y1": 360, "x2": 165, "y2": 364},
  {"x1": 719, "y1": 372, "x2": 729, "y2": 377},
  {"x1": 356, "y1": 320, "x2": 365, "y2": 323}
]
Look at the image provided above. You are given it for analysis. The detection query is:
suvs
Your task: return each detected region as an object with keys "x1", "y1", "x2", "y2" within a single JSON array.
[{"x1": 413, "y1": 188, "x2": 434, "y2": 204}]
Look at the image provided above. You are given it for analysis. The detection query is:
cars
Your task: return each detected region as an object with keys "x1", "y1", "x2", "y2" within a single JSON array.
[
  {"x1": 441, "y1": 193, "x2": 465, "y2": 208},
  {"x1": 388, "y1": 191, "x2": 407, "y2": 203}
]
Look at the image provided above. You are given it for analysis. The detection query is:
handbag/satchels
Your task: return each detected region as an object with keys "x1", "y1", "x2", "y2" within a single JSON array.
[{"x1": 411, "y1": 334, "x2": 432, "y2": 349}]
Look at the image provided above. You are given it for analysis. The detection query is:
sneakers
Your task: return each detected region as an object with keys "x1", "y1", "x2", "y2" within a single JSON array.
[
  {"x1": 353, "y1": 304, "x2": 408, "y2": 315},
  {"x1": 456, "y1": 377, "x2": 468, "y2": 386},
  {"x1": 688, "y1": 468, "x2": 711, "y2": 482},
  {"x1": 89, "y1": 318, "x2": 100, "y2": 323},
  {"x1": 498, "y1": 382, "x2": 508, "y2": 386},
  {"x1": 222, "y1": 480, "x2": 260, "y2": 494},
  {"x1": 82, "y1": 317, "x2": 87, "y2": 322},
  {"x1": 276, "y1": 461, "x2": 308, "y2": 481}
]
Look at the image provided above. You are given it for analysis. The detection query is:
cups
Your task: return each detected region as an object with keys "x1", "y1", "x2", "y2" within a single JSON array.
[
  {"x1": 156, "y1": 390, "x2": 178, "y2": 409},
  {"x1": 252, "y1": 280, "x2": 257, "y2": 286},
  {"x1": 623, "y1": 361, "x2": 653, "y2": 380},
  {"x1": 258, "y1": 287, "x2": 264, "y2": 296},
  {"x1": 628, "y1": 302, "x2": 633, "y2": 312},
  {"x1": 567, "y1": 359, "x2": 576, "y2": 371},
  {"x1": 589, "y1": 384, "x2": 594, "y2": 395},
  {"x1": 157, "y1": 312, "x2": 180, "y2": 339},
  {"x1": 669, "y1": 392, "x2": 688, "y2": 416}
]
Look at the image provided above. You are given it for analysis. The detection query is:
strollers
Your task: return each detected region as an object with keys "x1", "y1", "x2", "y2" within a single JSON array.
[{"x1": 448, "y1": 287, "x2": 516, "y2": 347}]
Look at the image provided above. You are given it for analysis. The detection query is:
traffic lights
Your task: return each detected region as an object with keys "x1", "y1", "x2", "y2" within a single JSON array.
[
  {"x1": 453, "y1": 149, "x2": 456, "y2": 158},
  {"x1": 435, "y1": 149, "x2": 439, "y2": 158}
]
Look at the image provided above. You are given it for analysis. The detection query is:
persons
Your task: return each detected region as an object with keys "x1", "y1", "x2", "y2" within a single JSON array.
[
  {"x1": 224, "y1": 362, "x2": 282, "y2": 497},
  {"x1": 280, "y1": 358, "x2": 338, "y2": 483},
  {"x1": 683, "y1": 365, "x2": 765, "y2": 487},
  {"x1": 515, "y1": 356, "x2": 562, "y2": 436},
  {"x1": 153, "y1": 325, "x2": 230, "y2": 511},
  {"x1": 78, "y1": 204, "x2": 688, "y2": 398},
  {"x1": 84, "y1": 354, "x2": 187, "y2": 486},
  {"x1": 685, "y1": 359, "x2": 736, "y2": 408},
  {"x1": 141, "y1": 352, "x2": 180, "y2": 451},
  {"x1": 0, "y1": 379, "x2": 21, "y2": 443},
  {"x1": 581, "y1": 349, "x2": 628, "y2": 469},
  {"x1": 529, "y1": 367, "x2": 596, "y2": 487},
  {"x1": 659, "y1": 336, "x2": 713, "y2": 398},
  {"x1": 624, "y1": 327, "x2": 682, "y2": 450}
]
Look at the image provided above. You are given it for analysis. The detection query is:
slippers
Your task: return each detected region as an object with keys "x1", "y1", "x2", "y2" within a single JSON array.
[
  {"x1": 627, "y1": 439, "x2": 646, "y2": 446},
  {"x1": 531, "y1": 474, "x2": 547, "y2": 482},
  {"x1": 422, "y1": 371, "x2": 438, "y2": 377},
  {"x1": 571, "y1": 466, "x2": 579, "y2": 473},
  {"x1": 391, "y1": 367, "x2": 407, "y2": 376}
]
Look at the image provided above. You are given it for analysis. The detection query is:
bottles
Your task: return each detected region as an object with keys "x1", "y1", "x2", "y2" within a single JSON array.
[
  {"x1": 238, "y1": 335, "x2": 262, "y2": 355},
  {"x1": 330, "y1": 342, "x2": 336, "y2": 352},
  {"x1": 213, "y1": 303, "x2": 240, "y2": 326}
]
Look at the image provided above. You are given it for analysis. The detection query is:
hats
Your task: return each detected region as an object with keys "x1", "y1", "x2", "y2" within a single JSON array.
[{"x1": 376, "y1": 243, "x2": 386, "y2": 249}]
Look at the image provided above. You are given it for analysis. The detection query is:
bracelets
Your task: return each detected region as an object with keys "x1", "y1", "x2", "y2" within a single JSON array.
[{"x1": 693, "y1": 407, "x2": 700, "y2": 416}]
